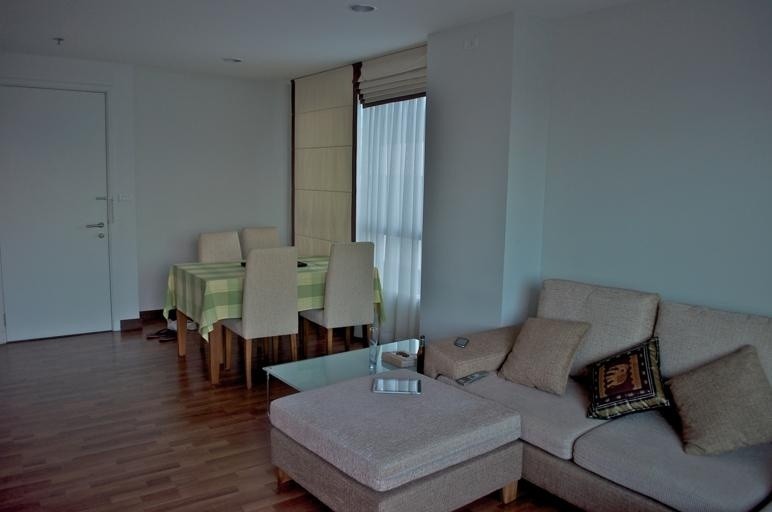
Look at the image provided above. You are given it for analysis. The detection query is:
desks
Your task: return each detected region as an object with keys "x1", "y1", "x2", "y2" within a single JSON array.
[{"x1": 262, "y1": 336, "x2": 421, "y2": 413}]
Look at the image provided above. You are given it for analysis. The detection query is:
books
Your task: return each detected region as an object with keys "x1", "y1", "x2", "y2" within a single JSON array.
[{"x1": 382, "y1": 349, "x2": 418, "y2": 368}]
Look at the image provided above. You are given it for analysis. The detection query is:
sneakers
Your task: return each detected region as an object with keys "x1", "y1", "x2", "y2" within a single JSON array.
[{"x1": 187, "y1": 319, "x2": 198, "y2": 331}]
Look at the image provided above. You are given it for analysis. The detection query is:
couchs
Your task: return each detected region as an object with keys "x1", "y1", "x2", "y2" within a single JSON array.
[{"x1": 424, "y1": 326, "x2": 772, "y2": 511}]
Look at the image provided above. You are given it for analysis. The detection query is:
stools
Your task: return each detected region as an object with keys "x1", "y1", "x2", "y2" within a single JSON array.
[{"x1": 268, "y1": 367, "x2": 523, "y2": 511}]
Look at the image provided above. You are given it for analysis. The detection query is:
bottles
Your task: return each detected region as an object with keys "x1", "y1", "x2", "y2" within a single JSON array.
[{"x1": 417, "y1": 335, "x2": 425, "y2": 374}]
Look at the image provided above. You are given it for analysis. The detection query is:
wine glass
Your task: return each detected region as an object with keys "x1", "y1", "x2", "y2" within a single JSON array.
[{"x1": 368, "y1": 325, "x2": 378, "y2": 370}]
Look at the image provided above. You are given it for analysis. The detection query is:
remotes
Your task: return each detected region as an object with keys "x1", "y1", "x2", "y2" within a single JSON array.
[{"x1": 456, "y1": 371, "x2": 489, "y2": 386}]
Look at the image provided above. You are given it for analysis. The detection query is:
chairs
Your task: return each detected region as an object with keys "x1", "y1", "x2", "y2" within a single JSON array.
[{"x1": 200, "y1": 224, "x2": 377, "y2": 391}]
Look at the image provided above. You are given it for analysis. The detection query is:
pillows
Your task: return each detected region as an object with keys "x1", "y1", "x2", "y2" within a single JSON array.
[
  {"x1": 537, "y1": 277, "x2": 659, "y2": 378},
  {"x1": 581, "y1": 336, "x2": 671, "y2": 419},
  {"x1": 497, "y1": 317, "x2": 592, "y2": 398},
  {"x1": 666, "y1": 342, "x2": 772, "y2": 455},
  {"x1": 652, "y1": 300, "x2": 772, "y2": 382}
]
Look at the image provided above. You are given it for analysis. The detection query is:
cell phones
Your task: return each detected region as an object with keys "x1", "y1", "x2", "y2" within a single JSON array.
[{"x1": 454, "y1": 337, "x2": 470, "y2": 348}]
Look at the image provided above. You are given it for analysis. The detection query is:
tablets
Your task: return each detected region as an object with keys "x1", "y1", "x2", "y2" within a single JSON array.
[{"x1": 371, "y1": 377, "x2": 421, "y2": 394}]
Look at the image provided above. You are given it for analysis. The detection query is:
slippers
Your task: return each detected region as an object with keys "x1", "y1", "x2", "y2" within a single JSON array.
[{"x1": 146, "y1": 328, "x2": 176, "y2": 342}]
